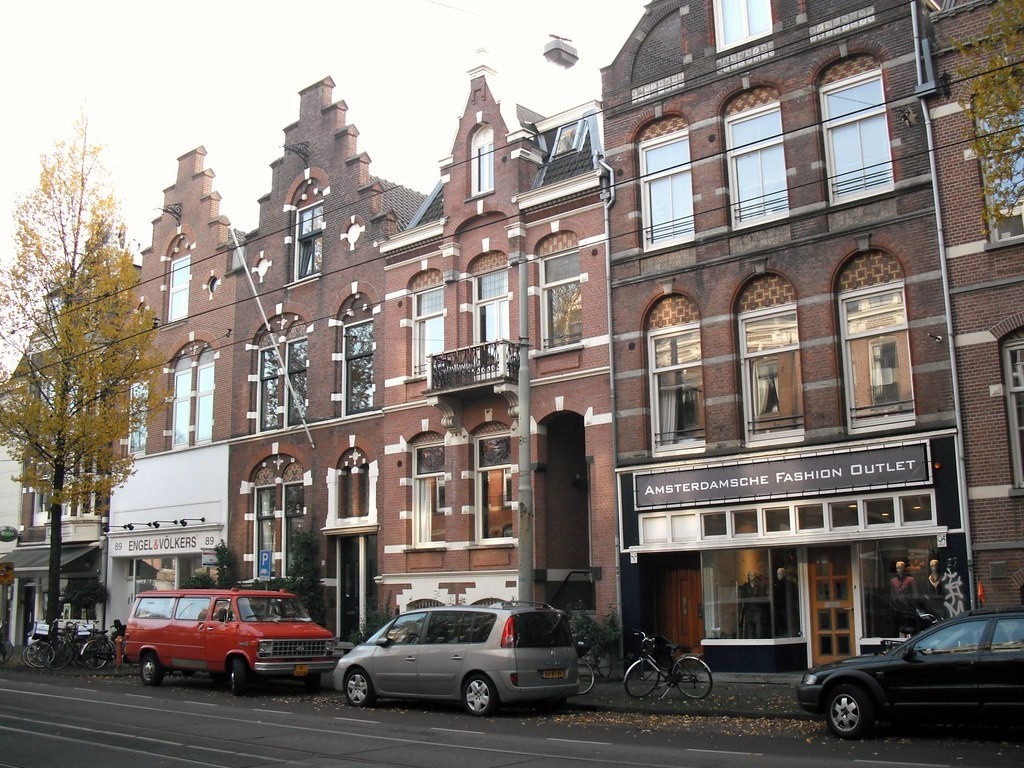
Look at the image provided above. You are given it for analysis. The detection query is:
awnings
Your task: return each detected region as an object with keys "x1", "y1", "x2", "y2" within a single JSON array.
[{"x1": 0, "y1": 546, "x2": 99, "y2": 578}]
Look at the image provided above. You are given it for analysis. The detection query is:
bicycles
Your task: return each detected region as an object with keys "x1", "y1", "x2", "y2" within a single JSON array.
[
  {"x1": 623, "y1": 629, "x2": 713, "y2": 700},
  {"x1": 21, "y1": 617, "x2": 139, "y2": 670},
  {"x1": 0, "y1": 618, "x2": 14, "y2": 664},
  {"x1": 574, "y1": 636, "x2": 613, "y2": 695}
]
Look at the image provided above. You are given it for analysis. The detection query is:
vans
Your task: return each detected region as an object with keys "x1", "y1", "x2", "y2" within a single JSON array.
[{"x1": 122, "y1": 587, "x2": 335, "y2": 695}]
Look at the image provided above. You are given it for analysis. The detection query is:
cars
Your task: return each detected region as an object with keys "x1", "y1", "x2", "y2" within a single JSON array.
[
  {"x1": 334, "y1": 602, "x2": 578, "y2": 715},
  {"x1": 797, "y1": 605, "x2": 1024, "y2": 736},
  {"x1": 862, "y1": 587, "x2": 947, "y2": 643}
]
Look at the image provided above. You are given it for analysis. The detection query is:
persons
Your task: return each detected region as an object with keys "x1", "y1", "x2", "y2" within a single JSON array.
[
  {"x1": 889, "y1": 561, "x2": 919, "y2": 639},
  {"x1": 772, "y1": 568, "x2": 798, "y2": 637},
  {"x1": 738, "y1": 571, "x2": 768, "y2": 639},
  {"x1": 926, "y1": 559, "x2": 949, "y2": 621}
]
